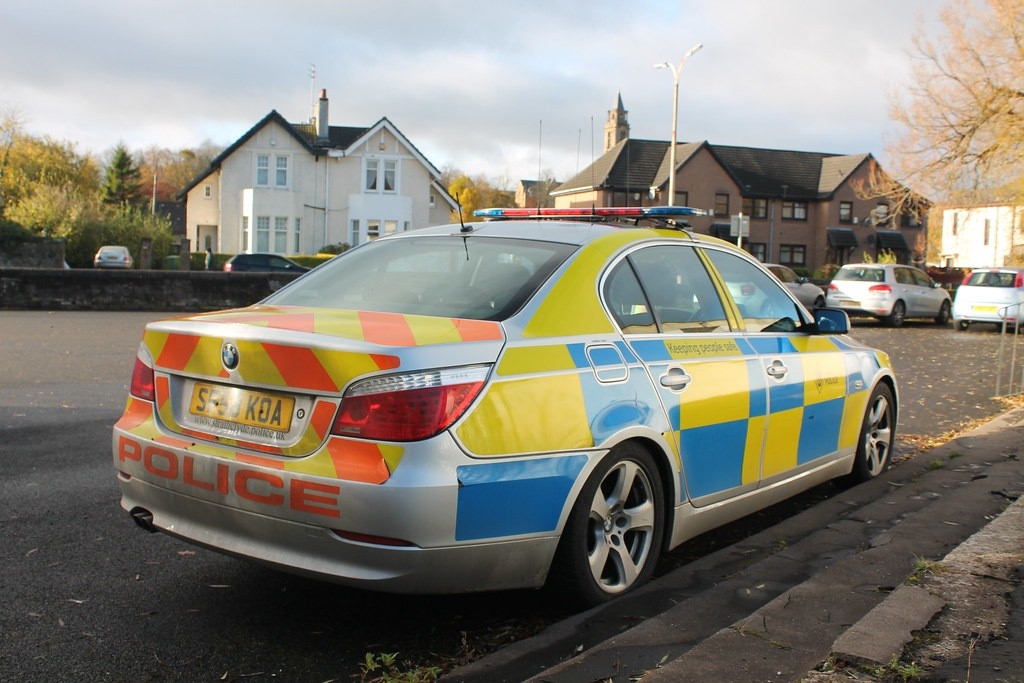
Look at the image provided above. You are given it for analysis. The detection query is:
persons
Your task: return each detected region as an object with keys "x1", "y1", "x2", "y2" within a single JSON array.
[
  {"x1": 927, "y1": 266, "x2": 938, "y2": 283},
  {"x1": 204, "y1": 247, "x2": 213, "y2": 271}
]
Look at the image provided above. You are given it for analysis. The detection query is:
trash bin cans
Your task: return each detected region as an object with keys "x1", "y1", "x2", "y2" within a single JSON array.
[{"x1": 167, "y1": 256, "x2": 181, "y2": 270}]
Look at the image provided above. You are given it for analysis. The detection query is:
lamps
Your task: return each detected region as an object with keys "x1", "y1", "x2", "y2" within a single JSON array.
[{"x1": 860, "y1": 217, "x2": 872, "y2": 225}]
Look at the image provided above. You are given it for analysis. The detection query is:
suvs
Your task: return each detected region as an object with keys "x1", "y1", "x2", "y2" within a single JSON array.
[{"x1": 222, "y1": 252, "x2": 313, "y2": 274}]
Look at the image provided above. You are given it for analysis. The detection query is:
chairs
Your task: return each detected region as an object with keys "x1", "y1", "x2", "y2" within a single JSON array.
[
  {"x1": 479, "y1": 264, "x2": 530, "y2": 308},
  {"x1": 898, "y1": 273, "x2": 909, "y2": 284},
  {"x1": 611, "y1": 266, "x2": 691, "y2": 326},
  {"x1": 985, "y1": 274, "x2": 1001, "y2": 286},
  {"x1": 864, "y1": 271, "x2": 880, "y2": 280}
]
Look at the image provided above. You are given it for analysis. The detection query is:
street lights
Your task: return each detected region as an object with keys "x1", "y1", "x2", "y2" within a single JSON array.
[{"x1": 654, "y1": 44, "x2": 703, "y2": 226}]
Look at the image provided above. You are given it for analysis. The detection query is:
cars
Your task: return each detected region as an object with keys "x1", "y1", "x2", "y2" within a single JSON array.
[
  {"x1": 950, "y1": 266, "x2": 1024, "y2": 335},
  {"x1": 826, "y1": 262, "x2": 954, "y2": 328},
  {"x1": 109, "y1": 207, "x2": 904, "y2": 609},
  {"x1": 93, "y1": 245, "x2": 134, "y2": 269},
  {"x1": 691, "y1": 261, "x2": 826, "y2": 318}
]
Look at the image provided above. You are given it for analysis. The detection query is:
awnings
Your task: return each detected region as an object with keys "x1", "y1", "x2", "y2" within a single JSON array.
[
  {"x1": 877, "y1": 230, "x2": 908, "y2": 258},
  {"x1": 716, "y1": 224, "x2": 749, "y2": 243},
  {"x1": 827, "y1": 229, "x2": 858, "y2": 256}
]
